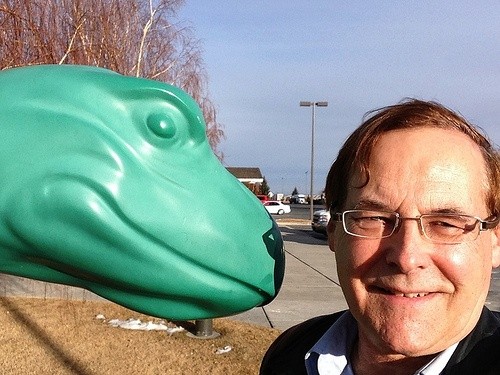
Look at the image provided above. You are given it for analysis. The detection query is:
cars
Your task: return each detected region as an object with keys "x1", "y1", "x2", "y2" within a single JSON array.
[
  {"x1": 289, "y1": 193, "x2": 327, "y2": 205},
  {"x1": 255, "y1": 195, "x2": 270, "y2": 205},
  {"x1": 311, "y1": 208, "x2": 331, "y2": 237},
  {"x1": 262, "y1": 201, "x2": 292, "y2": 215}
]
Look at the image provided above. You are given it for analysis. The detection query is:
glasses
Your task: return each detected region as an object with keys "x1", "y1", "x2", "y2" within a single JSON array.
[{"x1": 333, "y1": 208, "x2": 499, "y2": 245}]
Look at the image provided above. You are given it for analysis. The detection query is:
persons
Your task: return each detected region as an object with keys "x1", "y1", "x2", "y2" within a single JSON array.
[
  {"x1": 259, "y1": 97, "x2": 500, "y2": 375},
  {"x1": 266, "y1": 190, "x2": 274, "y2": 200}
]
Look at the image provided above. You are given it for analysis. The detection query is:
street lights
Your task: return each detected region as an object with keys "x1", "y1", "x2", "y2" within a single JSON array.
[{"x1": 299, "y1": 101, "x2": 328, "y2": 222}]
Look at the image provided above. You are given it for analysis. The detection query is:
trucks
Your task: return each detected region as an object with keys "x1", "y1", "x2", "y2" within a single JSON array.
[{"x1": 271, "y1": 193, "x2": 284, "y2": 205}]
